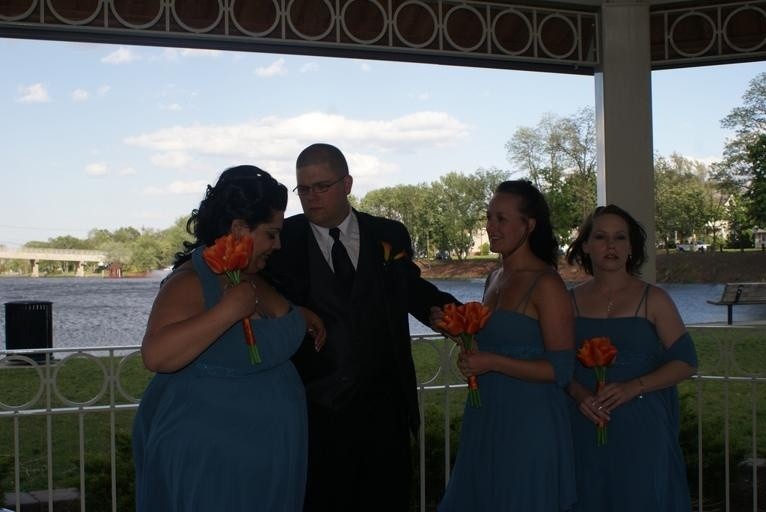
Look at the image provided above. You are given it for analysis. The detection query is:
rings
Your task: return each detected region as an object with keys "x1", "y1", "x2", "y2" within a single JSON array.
[
  {"x1": 255, "y1": 298, "x2": 259, "y2": 304},
  {"x1": 461, "y1": 361, "x2": 465, "y2": 368}
]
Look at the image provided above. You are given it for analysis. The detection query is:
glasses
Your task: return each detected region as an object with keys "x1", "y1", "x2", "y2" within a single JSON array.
[{"x1": 292, "y1": 174, "x2": 346, "y2": 195}]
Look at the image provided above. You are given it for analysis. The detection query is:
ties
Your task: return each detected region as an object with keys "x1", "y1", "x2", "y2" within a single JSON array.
[{"x1": 327, "y1": 227, "x2": 356, "y2": 291}]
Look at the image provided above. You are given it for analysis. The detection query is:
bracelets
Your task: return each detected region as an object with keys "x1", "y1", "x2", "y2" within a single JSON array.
[{"x1": 636, "y1": 376, "x2": 645, "y2": 400}]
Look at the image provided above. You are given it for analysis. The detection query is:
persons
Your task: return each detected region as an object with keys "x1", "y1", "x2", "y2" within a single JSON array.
[
  {"x1": 428, "y1": 178, "x2": 580, "y2": 512},
  {"x1": 252, "y1": 142, "x2": 475, "y2": 512},
  {"x1": 561, "y1": 202, "x2": 699, "y2": 511},
  {"x1": 129, "y1": 163, "x2": 329, "y2": 512}
]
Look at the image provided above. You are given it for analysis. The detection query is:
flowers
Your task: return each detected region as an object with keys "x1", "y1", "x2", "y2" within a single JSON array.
[
  {"x1": 577, "y1": 336, "x2": 618, "y2": 449},
  {"x1": 429, "y1": 301, "x2": 492, "y2": 409},
  {"x1": 202, "y1": 233, "x2": 263, "y2": 366}
]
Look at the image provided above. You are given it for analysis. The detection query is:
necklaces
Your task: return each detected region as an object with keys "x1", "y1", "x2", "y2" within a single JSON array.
[
  {"x1": 587, "y1": 274, "x2": 634, "y2": 313},
  {"x1": 494, "y1": 255, "x2": 541, "y2": 296},
  {"x1": 248, "y1": 278, "x2": 259, "y2": 289}
]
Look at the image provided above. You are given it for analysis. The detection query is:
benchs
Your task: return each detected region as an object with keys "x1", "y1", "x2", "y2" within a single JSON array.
[{"x1": 707, "y1": 281, "x2": 766, "y2": 325}]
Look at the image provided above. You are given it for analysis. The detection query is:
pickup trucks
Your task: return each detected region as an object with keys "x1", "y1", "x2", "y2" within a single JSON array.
[{"x1": 676, "y1": 239, "x2": 711, "y2": 253}]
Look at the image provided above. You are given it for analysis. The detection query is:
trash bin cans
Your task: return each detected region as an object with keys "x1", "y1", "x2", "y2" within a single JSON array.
[{"x1": 4, "y1": 301, "x2": 53, "y2": 365}]
